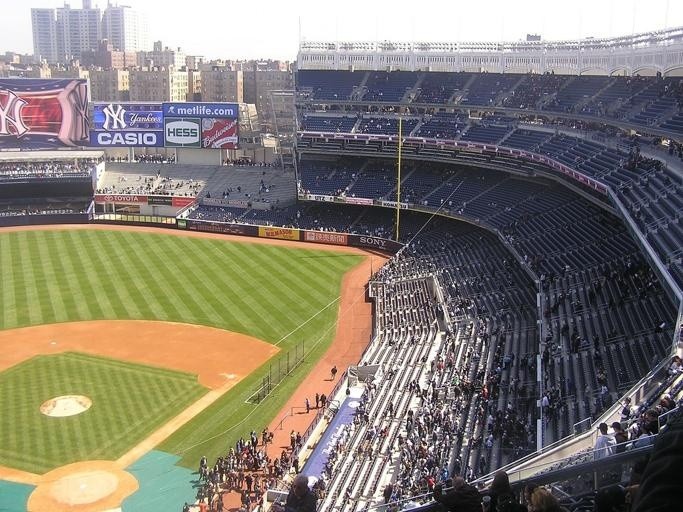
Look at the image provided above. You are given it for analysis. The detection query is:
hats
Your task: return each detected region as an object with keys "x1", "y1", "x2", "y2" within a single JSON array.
[
  {"x1": 597, "y1": 423, "x2": 608, "y2": 429},
  {"x1": 610, "y1": 422, "x2": 620, "y2": 428}
]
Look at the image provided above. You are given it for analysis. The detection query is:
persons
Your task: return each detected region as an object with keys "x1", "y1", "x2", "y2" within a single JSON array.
[
  {"x1": 285, "y1": 255, "x2": 682, "y2": 512},
  {"x1": 1, "y1": 67, "x2": 682, "y2": 254},
  {"x1": 183, "y1": 427, "x2": 302, "y2": 512}
]
